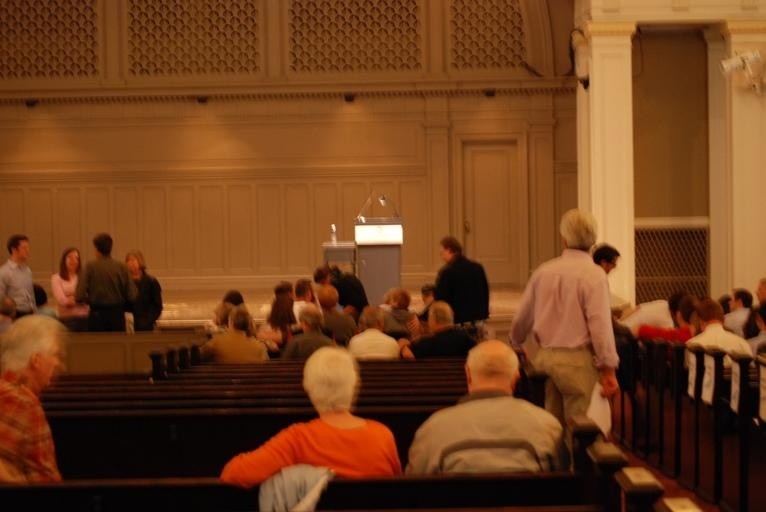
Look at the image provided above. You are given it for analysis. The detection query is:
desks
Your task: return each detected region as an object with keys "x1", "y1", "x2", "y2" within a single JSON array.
[
  {"x1": 319, "y1": 241, "x2": 355, "y2": 277},
  {"x1": 64, "y1": 322, "x2": 209, "y2": 376}
]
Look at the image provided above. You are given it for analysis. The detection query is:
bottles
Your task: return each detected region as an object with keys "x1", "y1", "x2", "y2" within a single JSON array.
[{"x1": 330, "y1": 223, "x2": 339, "y2": 244}]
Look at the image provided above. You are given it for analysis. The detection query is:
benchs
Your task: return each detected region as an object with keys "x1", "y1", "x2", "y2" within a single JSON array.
[
  {"x1": 609, "y1": 320, "x2": 765, "y2": 512},
  {"x1": 2, "y1": 359, "x2": 663, "y2": 511}
]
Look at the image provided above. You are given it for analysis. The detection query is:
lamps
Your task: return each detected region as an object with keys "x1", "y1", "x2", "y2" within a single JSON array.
[{"x1": 716, "y1": 45, "x2": 763, "y2": 97}]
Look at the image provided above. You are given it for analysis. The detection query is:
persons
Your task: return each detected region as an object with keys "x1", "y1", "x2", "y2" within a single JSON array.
[
  {"x1": 0, "y1": 296, "x2": 17, "y2": 325},
  {"x1": 404, "y1": 338, "x2": 573, "y2": 475},
  {"x1": 508, "y1": 208, "x2": 620, "y2": 425},
  {"x1": 0, "y1": 233, "x2": 38, "y2": 321},
  {"x1": 50, "y1": 246, "x2": 89, "y2": 332},
  {"x1": 73, "y1": 232, "x2": 140, "y2": 332},
  {"x1": 592, "y1": 242, "x2": 766, "y2": 422},
  {"x1": 0, "y1": 312, "x2": 63, "y2": 484},
  {"x1": 219, "y1": 343, "x2": 403, "y2": 490},
  {"x1": 124, "y1": 248, "x2": 163, "y2": 331},
  {"x1": 33, "y1": 283, "x2": 56, "y2": 319}
]
